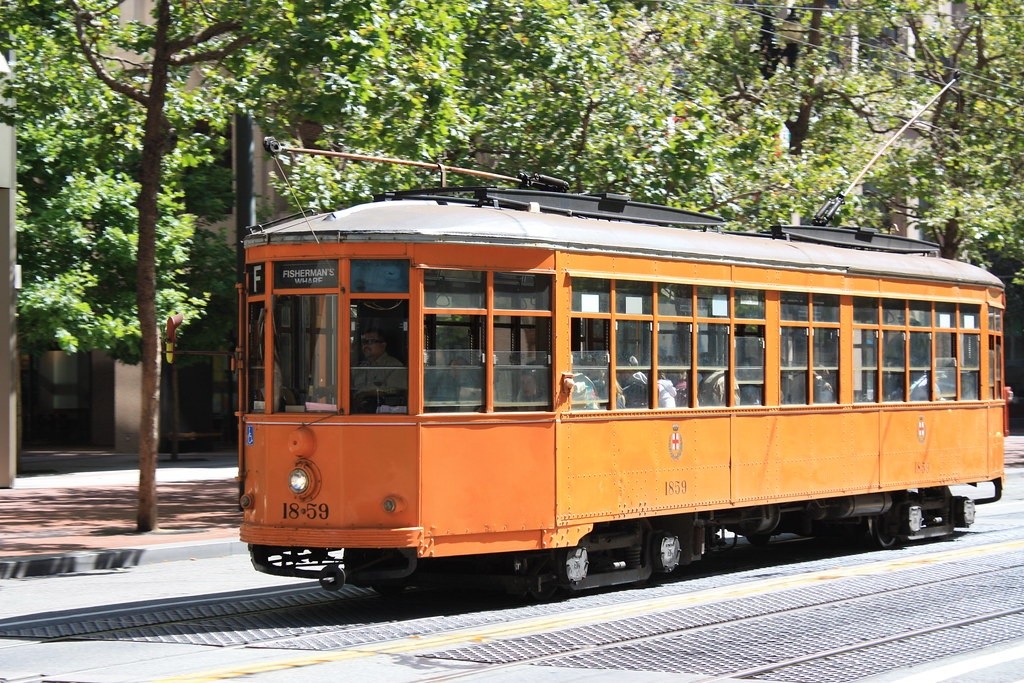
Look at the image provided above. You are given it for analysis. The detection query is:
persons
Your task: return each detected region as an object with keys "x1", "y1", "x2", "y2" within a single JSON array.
[
  {"x1": 350, "y1": 328, "x2": 407, "y2": 389},
  {"x1": 516, "y1": 352, "x2": 978, "y2": 409}
]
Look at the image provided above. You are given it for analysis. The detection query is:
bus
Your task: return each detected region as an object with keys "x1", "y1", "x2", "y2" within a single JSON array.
[{"x1": 234, "y1": 78, "x2": 1015, "y2": 611}]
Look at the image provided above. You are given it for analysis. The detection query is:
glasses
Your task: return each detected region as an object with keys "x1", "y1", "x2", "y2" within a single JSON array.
[{"x1": 361, "y1": 339, "x2": 383, "y2": 345}]
